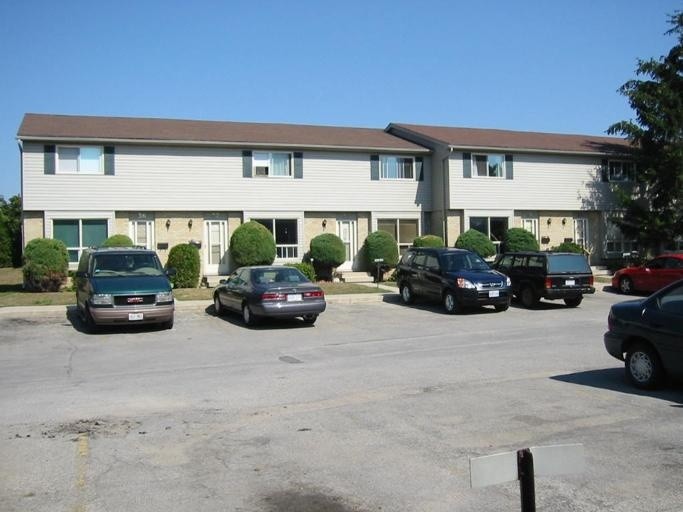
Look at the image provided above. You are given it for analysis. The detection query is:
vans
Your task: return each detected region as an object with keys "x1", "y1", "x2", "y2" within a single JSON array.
[{"x1": 76, "y1": 245, "x2": 174, "y2": 329}]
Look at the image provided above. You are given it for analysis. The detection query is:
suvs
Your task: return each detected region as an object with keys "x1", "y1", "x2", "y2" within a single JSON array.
[{"x1": 397, "y1": 247, "x2": 595, "y2": 314}]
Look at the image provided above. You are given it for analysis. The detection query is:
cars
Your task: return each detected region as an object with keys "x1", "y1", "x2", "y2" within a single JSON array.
[
  {"x1": 213, "y1": 265, "x2": 326, "y2": 326},
  {"x1": 604, "y1": 254, "x2": 683, "y2": 388}
]
